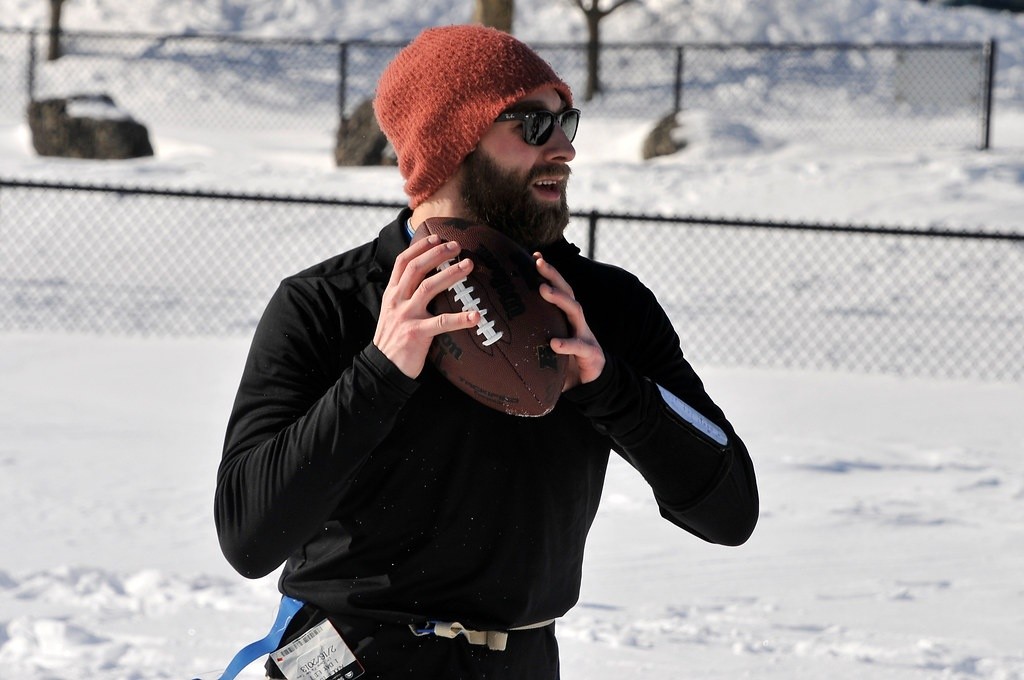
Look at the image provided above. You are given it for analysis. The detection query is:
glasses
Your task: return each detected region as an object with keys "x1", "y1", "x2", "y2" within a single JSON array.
[{"x1": 493, "y1": 108, "x2": 581, "y2": 146}]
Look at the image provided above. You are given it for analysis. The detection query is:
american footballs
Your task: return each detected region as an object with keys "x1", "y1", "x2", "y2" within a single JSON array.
[{"x1": 407, "y1": 217, "x2": 569, "y2": 416}]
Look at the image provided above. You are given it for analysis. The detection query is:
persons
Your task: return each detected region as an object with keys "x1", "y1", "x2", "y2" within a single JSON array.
[{"x1": 213, "y1": 23, "x2": 761, "y2": 680}]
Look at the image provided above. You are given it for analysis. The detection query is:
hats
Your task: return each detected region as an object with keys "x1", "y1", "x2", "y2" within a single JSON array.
[{"x1": 373, "y1": 24, "x2": 573, "y2": 210}]
